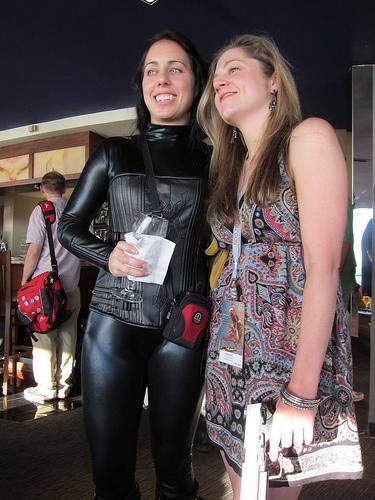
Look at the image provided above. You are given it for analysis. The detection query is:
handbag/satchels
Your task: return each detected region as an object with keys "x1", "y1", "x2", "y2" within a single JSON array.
[
  {"x1": 15, "y1": 272, "x2": 74, "y2": 342},
  {"x1": 162, "y1": 292, "x2": 211, "y2": 352}
]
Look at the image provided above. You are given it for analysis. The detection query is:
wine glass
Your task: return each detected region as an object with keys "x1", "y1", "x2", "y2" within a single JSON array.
[
  {"x1": 112, "y1": 211, "x2": 168, "y2": 303},
  {"x1": 0, "y1": 242, "x2": 8, "y2": 252}
]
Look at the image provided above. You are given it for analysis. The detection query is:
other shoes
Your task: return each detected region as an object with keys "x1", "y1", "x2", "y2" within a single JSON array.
[
  {"x1": 57, "y1": 392, "x2": 71, "y2": 400},
  {"x1": 24, "y1": 386, "x2": 54, "y2": 402}
]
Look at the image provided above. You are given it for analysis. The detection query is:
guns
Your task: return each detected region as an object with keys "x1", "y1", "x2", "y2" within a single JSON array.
[{"x1": 240, "y1": 402, "x2": 284, "y2": 500}]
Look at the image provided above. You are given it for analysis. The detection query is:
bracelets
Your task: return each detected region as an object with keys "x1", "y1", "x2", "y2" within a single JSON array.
[{"x1": 280, "y1": 381, "x2": 323, "y2": 410}]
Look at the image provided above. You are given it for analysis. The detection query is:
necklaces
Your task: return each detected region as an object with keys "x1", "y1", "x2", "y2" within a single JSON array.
[{"x1": 239, "y1": 152, "x2": 252, "y2": 192}]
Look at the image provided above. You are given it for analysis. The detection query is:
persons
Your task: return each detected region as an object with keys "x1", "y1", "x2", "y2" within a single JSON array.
[
  {"x1": 57, "y1": 31, "x2": 218, "y2": 500},
  {"x1": 196, "y1": 36, "x2": 363, "y2": 500},
  {"x1": 338, "y1": 196, "x2": 364, "y2": 402},
  {"x1": 361, "y1": 217, "x2": 372, "y2": 298},
  {"x1": 21, "y1": 171, "x2": 81, "y2": 403}
]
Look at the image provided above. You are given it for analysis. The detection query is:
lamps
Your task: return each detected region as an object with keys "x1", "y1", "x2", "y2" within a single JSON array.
[{"x1": 141, "y1": 0, "x2": 157, "y2": 5}]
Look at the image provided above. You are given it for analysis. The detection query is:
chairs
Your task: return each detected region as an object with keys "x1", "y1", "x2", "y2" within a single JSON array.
[{"x1": 0, "y1": 250, "x2": 32, "y2": 396}]
